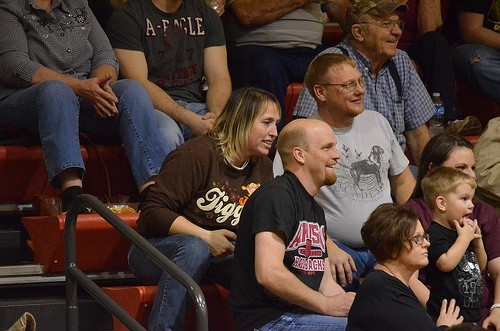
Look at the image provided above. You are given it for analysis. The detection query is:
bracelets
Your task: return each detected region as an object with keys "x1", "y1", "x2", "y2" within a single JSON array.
[{"x1": 490, "y1": 303, "x2": 500, "y2": 313}]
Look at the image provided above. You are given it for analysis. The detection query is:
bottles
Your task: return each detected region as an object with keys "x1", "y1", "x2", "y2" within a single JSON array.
[{"x1": 429, "y1": 93, "x2": 444, "y2": 136}]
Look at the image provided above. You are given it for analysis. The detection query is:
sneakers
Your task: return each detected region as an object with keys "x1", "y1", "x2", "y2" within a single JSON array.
[{"x1": 136, "y1": 183, "x2": 155, "y2": 214}]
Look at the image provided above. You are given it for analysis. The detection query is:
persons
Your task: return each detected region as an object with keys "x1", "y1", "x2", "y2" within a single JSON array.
[
  {"x1": 231, "y1": 119, "x2": 357, "y2": 330},
  {"x1": 0, "y1": 0, "x2": 500, "y2": 331}
]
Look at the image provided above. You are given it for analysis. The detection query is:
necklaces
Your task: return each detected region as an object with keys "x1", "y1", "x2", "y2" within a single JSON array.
[{"x1": 381, "y1": 263, "x2": 395, "y2": 275}]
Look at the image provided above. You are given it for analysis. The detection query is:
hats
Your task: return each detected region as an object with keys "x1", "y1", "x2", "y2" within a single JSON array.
[{"x1": 345, "y1": 0, "x2": 408, "y2": 27}]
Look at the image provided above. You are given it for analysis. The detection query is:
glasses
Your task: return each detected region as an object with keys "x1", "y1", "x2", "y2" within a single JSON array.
[
  {"x1": 402, "y1": 233, "x2": 430, "y2": 244},
  {"x1": 316, "y1": 76, "x2": 366, "y2": 92},
  {"x1": 355, "y1": 19, "x2": 403, "y2": 29}
]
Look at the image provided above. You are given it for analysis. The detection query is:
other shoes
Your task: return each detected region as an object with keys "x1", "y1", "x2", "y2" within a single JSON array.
[{"x1": 60, "y1": 186, "x2": 93, "y2": 215}]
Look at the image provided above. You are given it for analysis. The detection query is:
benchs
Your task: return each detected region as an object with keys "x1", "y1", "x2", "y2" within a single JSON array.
[{"x1": 0, "y1": 23, "x2": 482, "y2": 331}]
